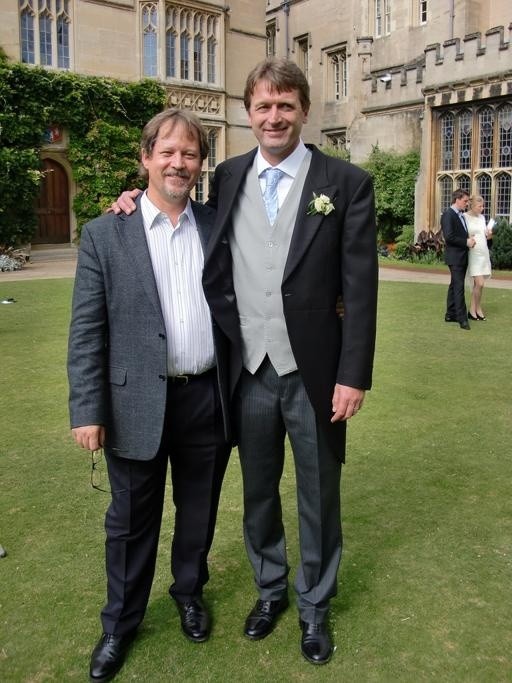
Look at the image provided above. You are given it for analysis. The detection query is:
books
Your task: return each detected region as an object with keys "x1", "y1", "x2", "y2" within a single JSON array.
[{"x1": 487, "y1": 218, "x2": 497, "y2": 229}]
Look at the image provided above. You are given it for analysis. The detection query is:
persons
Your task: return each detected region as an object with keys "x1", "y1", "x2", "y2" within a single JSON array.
[
  {"x1": 101, "y1": 55, "x2": 382, "y2": 668},
  {"x1": 434, "y1": 187, "x2": 473, "y2": 330},
  {"x1": 66, "y1": 107, "x2": 235, "y2": 682},
  {"x1": 460, "y1": 194, "x2": 494, "y2": 322}
]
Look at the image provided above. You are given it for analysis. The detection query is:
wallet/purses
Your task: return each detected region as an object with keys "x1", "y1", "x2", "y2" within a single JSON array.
[{"x1": 487, "y1": 219, "x2": 496, "y2": 230}]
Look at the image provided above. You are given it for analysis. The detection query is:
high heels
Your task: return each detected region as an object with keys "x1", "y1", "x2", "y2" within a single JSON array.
[{"x1": 468, "y1": 311, "x2": 486, "y2": 321}]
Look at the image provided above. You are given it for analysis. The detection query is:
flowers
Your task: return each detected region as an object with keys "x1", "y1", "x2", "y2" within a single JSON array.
[{"x1": 306, "y1": 192, "x2": 336, "y2": 215}]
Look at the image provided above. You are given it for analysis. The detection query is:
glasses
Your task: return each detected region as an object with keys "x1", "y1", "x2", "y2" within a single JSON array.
[{"x1": 91, "y1": 445, "x2": 129, "y2": 495}]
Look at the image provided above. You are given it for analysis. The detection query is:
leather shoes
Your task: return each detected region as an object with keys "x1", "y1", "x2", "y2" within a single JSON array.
[
  {"x1": 445, "y1": 314, "x2": 470, "y2": 330},
  {"x1": 176, "y1": 598, "x2": 211, "y2": 642},
  {"x1": 244, "y1": 596, "x2": 289, "y2": 639},
  {"x1": 89, "y1": 632, "x2": 138, "y2": 683},
  {"x1": 298, "y1": 616, "x2": 334, "y2": 664}
]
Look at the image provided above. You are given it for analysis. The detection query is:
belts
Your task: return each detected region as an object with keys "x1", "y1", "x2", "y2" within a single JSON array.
[{"x1": 167, "y1": 367, "x2": 217, "y2": 387}]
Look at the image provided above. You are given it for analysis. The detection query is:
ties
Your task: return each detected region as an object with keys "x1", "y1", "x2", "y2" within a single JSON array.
[
  {"x1": 263, "y1": 167, "x2": 284, "y2": 225},
  {"x1": 461, "y1": 216, "x2": 468, "y2": 233}
]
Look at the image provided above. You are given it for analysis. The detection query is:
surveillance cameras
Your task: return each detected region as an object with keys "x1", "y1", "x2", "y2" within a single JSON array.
[{"x1": 380, "y1": 77, "x2": 392, "y2": 83}]
